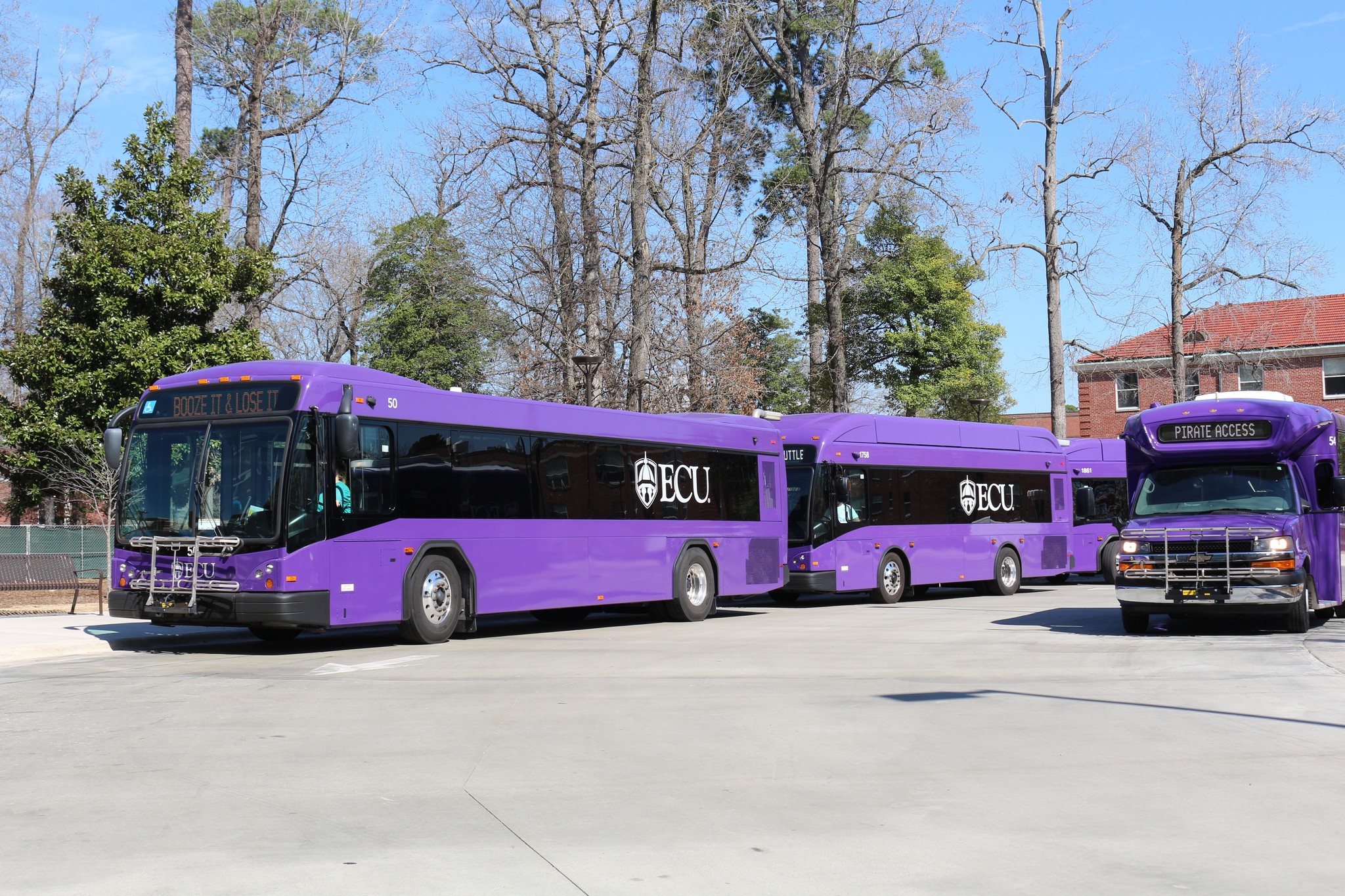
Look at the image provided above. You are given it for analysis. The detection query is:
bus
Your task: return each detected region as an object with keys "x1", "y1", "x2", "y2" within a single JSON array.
[
  {"x1": 103, "y1": 360, "x2": 790, "y2": 645},
  {"x1": 1058, "y1": 438, "x2": 1129, "y2": 585},
  {"x1": 767, "y1": 413, "x2": 1075, "y2": 604}
]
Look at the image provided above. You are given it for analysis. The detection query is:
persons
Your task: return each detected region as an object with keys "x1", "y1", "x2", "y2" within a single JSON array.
[
  {"x1": 821, "y1": 494, "x2": 861, "y2": 528},
  {"x1": 1264, "y1": 472, "x2": 1286, "y2": 498},
  {"x1": 310, "y1": 461, "x2": 353, "y2": 531}
]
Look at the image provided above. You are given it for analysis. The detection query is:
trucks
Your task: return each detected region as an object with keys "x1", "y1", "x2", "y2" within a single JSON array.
[{"x1": 1076, "y1": 391, "x2": 1345, "y2": 637}]
